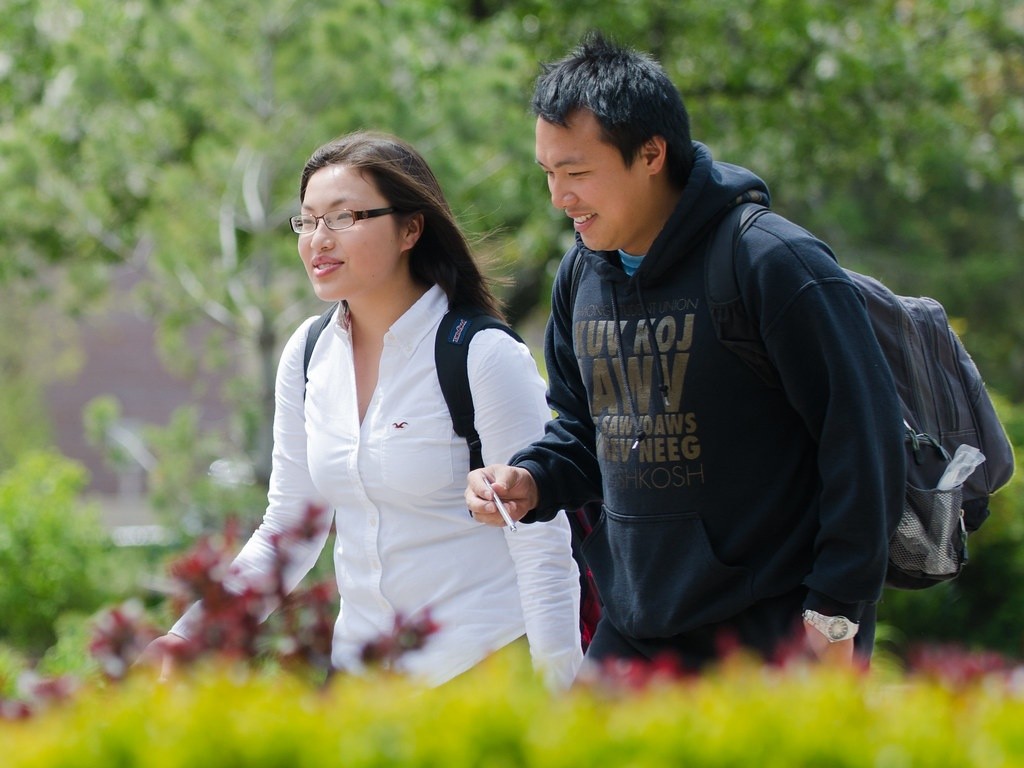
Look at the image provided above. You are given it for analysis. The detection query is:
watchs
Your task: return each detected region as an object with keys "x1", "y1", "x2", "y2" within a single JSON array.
[{"x1": 802, "y1": 609, "x2": 861, "y2": 644}]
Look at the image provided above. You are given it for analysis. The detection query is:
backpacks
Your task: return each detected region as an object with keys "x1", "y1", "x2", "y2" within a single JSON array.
[{"x1": 570, "y1": 203, "x2": 1014, "y2": 593}]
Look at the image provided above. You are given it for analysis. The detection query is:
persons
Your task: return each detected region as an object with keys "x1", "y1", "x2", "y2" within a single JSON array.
[
  {"x1": 124, "y1": 127, "x2": 585, "y2": 709},
  {"x1": 464, "y1": 27, "x2": 909, "y2": 695}
]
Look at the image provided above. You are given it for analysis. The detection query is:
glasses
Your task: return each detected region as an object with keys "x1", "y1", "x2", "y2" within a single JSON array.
[{"x1": 289, "y1": 206, "x2": 400, "y2": 234}]
